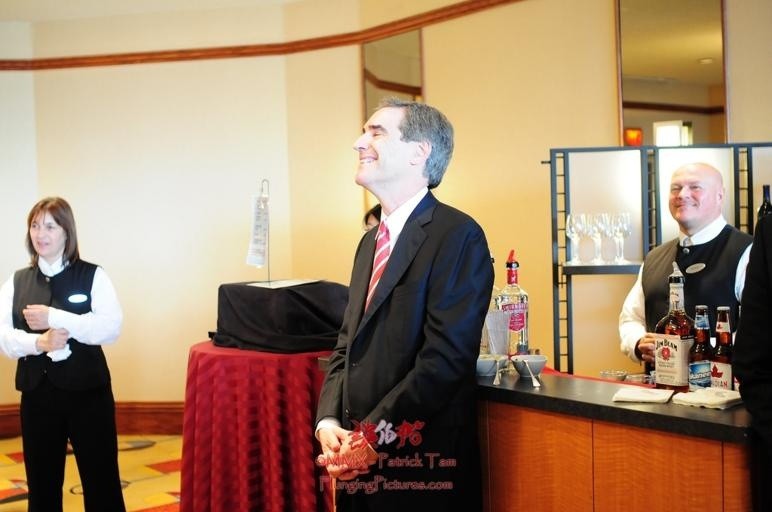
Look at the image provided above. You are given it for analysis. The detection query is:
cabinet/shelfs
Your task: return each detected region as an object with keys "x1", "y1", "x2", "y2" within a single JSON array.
[{"x1": 538, "y1": 145, "x2": 772, "y2": 378}]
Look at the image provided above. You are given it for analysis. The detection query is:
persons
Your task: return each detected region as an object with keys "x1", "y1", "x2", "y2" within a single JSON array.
[
  {"x1": 619, "y1": 163, "x2": 754, "y2": 389},
  {"x1": 313, "y1": 95, "x2": 494, "y2": 512},
  {"x1": 0, "y1": 196, "x2": 126, "y2": 512},
  {"x1": 731, "y1": 213, "x2": 772, "y2": 512},
  {"x1": 363, "y1": 203, "x2": 381, "y2": 232}
]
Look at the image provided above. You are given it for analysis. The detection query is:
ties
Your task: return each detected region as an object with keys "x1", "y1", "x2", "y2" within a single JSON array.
[{"x1": 364, "y1": 220, "x2": 390, "y2": 313}]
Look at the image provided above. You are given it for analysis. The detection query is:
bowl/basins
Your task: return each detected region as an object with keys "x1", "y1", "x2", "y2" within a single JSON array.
[
  {"x1": 515, "y1": 378, "x2": 545, "y2": 395},
  {"x1": 476, "y1": 354, "x2": 547, "y2": 378}
]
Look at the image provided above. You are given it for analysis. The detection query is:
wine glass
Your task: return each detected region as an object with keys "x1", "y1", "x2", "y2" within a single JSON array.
[{"x1": 566, "y1": 214, "x2": 634, "y2": 267}]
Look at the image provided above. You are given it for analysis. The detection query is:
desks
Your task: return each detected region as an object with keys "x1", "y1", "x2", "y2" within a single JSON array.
[{"x1": 181, "y1": 343, "x2": 335, "y2": 512}]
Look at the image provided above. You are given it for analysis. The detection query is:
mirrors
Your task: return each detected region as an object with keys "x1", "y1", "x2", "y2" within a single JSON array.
[{"x1": 613, "y1": 0, "x2": 730, "y2": 147}]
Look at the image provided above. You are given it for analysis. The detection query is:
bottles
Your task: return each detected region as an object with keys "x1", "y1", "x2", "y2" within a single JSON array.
[
  {"x1": 757, "y1": 184, "x2": 772, "y2": 223},
  {"x1": 479, "y1": 258, "x2": 529, "y2": 374},
  {"x1": 653, "y1": 271, "x2": 734, "y2": 392}
]
